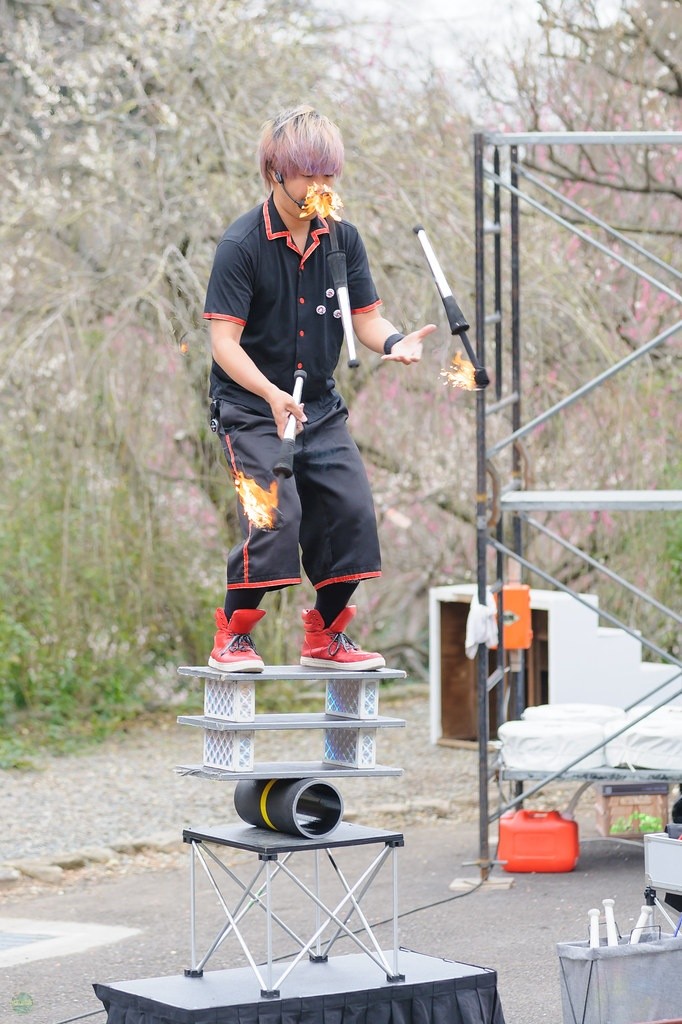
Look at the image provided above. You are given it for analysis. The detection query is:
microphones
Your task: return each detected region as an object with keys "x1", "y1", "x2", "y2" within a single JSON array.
[{"x1": 274, "y1": 171, "x2": 310, "y2": 210}]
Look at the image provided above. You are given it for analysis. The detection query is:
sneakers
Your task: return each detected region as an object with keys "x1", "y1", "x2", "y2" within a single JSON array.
[
  {"x1": 299, "y1": 605, "x2": 386, "y2": 670},
  {"x1": 211, "y1": 607, "x2": 264, "y2": 675}
]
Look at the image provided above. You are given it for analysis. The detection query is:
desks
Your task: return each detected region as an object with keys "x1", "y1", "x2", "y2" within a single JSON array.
[{"x1": 93, "y1": 948, "x2": 504, "y2": 1024}]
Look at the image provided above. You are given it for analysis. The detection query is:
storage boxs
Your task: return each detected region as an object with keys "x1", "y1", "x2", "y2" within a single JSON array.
[
  {"x1": 498, "y1": 808, "x2": 579, "y2": 871},
  {"x1": 597, "y1": 780, "x2": 668, "y2": 836},
  {"x1": 556, "y1": 931, "x2": 682, "y2": 1024}
]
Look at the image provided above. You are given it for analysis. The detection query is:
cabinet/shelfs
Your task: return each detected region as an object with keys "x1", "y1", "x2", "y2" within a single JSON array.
[{"x1": 473, "y1": 131, "x2": 681, "y2": 876}]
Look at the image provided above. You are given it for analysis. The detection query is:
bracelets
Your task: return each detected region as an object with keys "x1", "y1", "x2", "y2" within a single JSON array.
[{"x1": 383, "y1": 333, "x2": 405, "y2": 356}]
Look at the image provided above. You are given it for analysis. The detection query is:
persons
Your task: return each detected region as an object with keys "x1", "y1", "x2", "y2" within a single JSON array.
[{"x1": 200, "y1": 104, "x2": 438, "y2": 672}]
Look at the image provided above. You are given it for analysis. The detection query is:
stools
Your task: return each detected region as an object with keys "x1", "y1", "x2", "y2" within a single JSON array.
[{"x1": 183, "y1": 812, "x2": 405, "y2": 996}]
